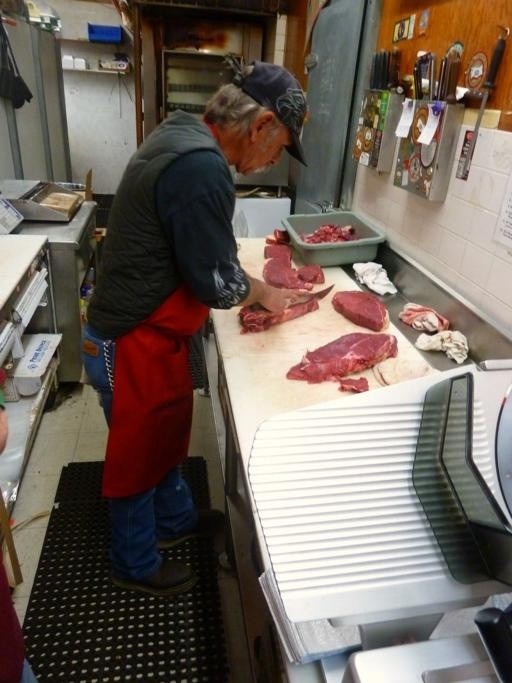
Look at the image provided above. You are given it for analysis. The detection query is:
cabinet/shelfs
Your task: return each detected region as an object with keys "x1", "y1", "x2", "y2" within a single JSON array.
[
  {"x1": 162, "y1": 49, "x2": 244, "y2": 121},
  {"x1": 0, "y1": 234, "x2": 62, "y2": 584},
  {"x1": 61, "y1": 35, "x2": 130, "y2": 76}
]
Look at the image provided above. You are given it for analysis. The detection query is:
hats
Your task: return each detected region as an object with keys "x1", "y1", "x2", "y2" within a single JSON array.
[{"x1": 232, "y1": 61, "x2": 309, "y2": 169}]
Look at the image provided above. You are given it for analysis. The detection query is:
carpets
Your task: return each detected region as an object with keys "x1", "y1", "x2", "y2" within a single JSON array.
[{"x1": 20, "y1": 458, "x2": 230, "y2": 683}]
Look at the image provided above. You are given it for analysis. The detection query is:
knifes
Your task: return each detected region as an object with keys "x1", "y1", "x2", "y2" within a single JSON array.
[{"x1": 247, "y1": 279, "x2": 336, "y2": 312}]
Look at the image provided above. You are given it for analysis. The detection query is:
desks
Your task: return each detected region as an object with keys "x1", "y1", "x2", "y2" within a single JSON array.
[{"x1": 203, "y1": 238, "x2": 512, "y2": 683}]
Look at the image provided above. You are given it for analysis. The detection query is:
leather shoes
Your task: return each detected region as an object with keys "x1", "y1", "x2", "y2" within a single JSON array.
[
  {"x1": 159, "y1": 509, "x2": 226, "y2": 548},
  {"x1": 112, "y1": 560, "x2": 197, "y2": 594}
]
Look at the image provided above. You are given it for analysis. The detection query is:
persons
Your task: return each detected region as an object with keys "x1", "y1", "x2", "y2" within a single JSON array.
[
  {"x1": 82, "y1": 60, "x2": 308, "y2": 596},
  {"x1": 0, "y1": 391, "x2": 37, "y2": 683}
]
